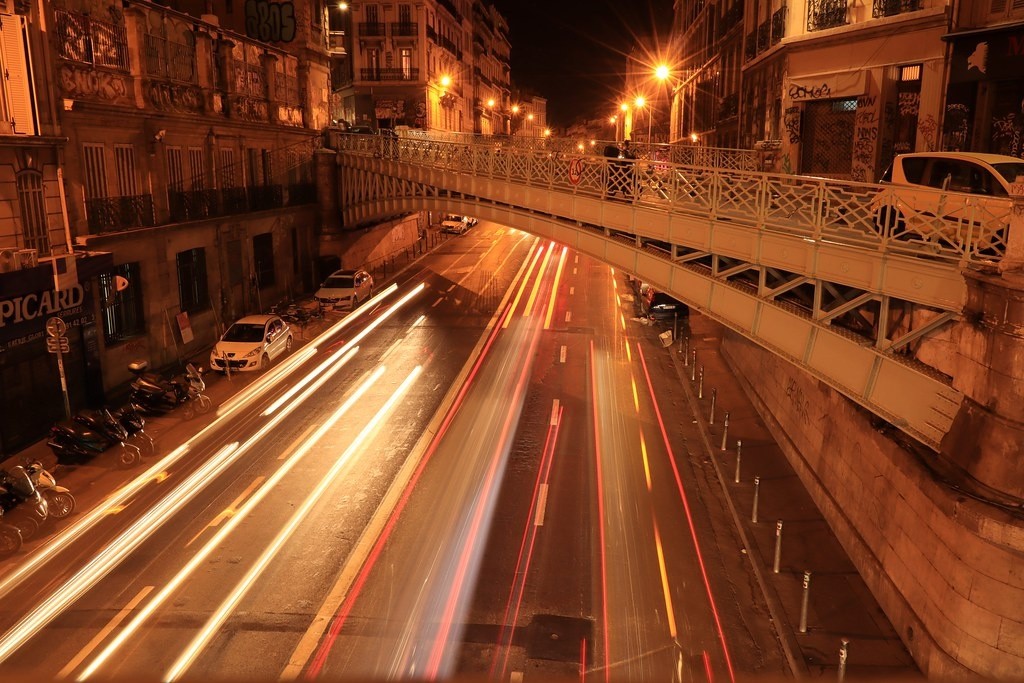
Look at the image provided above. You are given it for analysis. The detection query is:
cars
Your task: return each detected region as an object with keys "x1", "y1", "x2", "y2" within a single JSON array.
[
  {"x1": 441, "y1": 213, "x2": 469, "y2": 235},
  {"x1": 463, "y1": 216, "x2": 478, "y2": 228},
  {"x1": 210, "y1": 314, "x2": 294, "y2": 375},
  {"x1": 872, "y1": 151, "x2": 1024, "y2": 257},
  {"x1": 633, "y1": 277, "x2": 690, "y2": 326},
  {"x1": 313, "y1": 269, "x2": 373, "y2": 313}
]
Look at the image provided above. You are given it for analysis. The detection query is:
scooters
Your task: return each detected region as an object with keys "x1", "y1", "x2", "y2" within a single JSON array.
[
  {"x1": 602, "y1": 138, "x2": 643, "y2": 204},
  {"x1": 0, "y1": 359, "x2": 213, "y2": 561}
]
